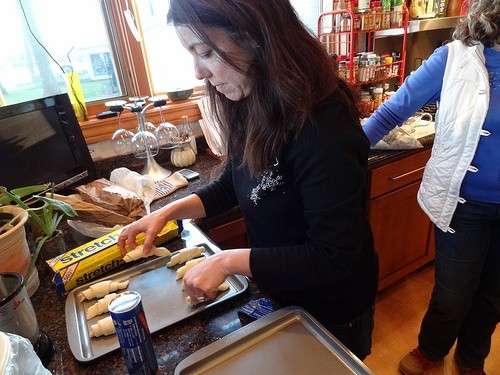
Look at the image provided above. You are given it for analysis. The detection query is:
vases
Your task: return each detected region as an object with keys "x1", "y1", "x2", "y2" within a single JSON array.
[
  {"x1": 0, "y1": 272, "x2": 40, "y2": 347},
  {"x1": 0, "y1": 206, "x2": 40, "y2": 297}
]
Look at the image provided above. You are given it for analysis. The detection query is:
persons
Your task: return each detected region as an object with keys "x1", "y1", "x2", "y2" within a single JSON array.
[
  {"x1": 361, "y1": 0, "x2": 500, "y2": 375},
  {"x1": 118, "y1": 0, "x2": 379, "y2": 360}
]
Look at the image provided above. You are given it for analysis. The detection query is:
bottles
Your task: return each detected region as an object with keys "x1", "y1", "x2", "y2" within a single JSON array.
[
  {"x1": 328, "y1": 51, "x2": 399, "y2": 112},
  {"x1": 336, "y1": 0, "x2": 463, "y2": 34},
  {"x1": 181, "y1": 115, "x2": 198, "y2": 155}
]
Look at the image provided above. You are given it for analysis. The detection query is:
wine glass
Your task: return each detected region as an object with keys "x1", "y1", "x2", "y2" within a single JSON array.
[{"x1": 104, "y1": 95, "x2": 179, "y2": 159}]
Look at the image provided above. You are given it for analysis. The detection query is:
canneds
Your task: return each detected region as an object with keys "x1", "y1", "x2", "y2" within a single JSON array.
[
  {"x1": 351, "y1": 0, "x2": 404, "y2": 31},
  {"x1": 337, "y1": 51, "x2": 399, "y2": 117},
  {"x1": 109, "y1": 290, "x2": 158, "y2": 375}
]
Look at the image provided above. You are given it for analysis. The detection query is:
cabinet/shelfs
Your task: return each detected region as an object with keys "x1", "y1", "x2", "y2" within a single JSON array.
[
  {"x1": 205, "y1": 143, "x2": 437, "y2": 297},
  {"x1": 316, "y1": 6, "x2": 409, "y2": 121}
]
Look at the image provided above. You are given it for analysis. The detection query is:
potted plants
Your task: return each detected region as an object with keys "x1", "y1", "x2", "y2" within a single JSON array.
[{"x1": 0, "y1": 187, "x2": 80, "y2": 290}]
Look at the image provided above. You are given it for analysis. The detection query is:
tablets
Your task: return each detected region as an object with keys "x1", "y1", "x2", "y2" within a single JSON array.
[{"x1": 0, "y1": 94, "x2": 98, "y2": 210}]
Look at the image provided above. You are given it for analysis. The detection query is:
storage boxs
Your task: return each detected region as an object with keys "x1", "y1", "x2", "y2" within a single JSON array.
[{"x1": 47, "y1": 217, "x2": 178, "y2": 296}]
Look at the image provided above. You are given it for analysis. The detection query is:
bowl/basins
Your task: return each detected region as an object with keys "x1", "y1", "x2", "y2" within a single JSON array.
[{"x1": 167, "y1": 88, "x2": 193, "y2": 102}]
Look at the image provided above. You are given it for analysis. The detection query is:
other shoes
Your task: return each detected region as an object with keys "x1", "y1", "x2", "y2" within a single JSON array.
[
  {"x1": 398, "y1": 348, "x2": 440, "y2": 373},
  {"x1": 455, "y1": 358, "x2": 487, "y2": 375}
]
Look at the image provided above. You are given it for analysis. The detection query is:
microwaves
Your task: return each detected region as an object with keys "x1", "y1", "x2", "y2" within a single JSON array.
[{"x1": 0, "y1": 93, "x2": 96, "y2": 209}]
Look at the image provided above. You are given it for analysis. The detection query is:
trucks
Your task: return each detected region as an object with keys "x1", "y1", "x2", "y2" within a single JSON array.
[{"x1": 57, "y1": 48, "x2": 116, "y2": 84}]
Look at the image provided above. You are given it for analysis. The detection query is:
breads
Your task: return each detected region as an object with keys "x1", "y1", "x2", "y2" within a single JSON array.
[{"x1": 46, "y1": 166, "x2": 155, "y2": 228}]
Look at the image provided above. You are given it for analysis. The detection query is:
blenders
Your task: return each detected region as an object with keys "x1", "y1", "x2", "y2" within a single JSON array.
[{"x1": 0, "y1": 271, "x2": 55, "y2": 367}]
